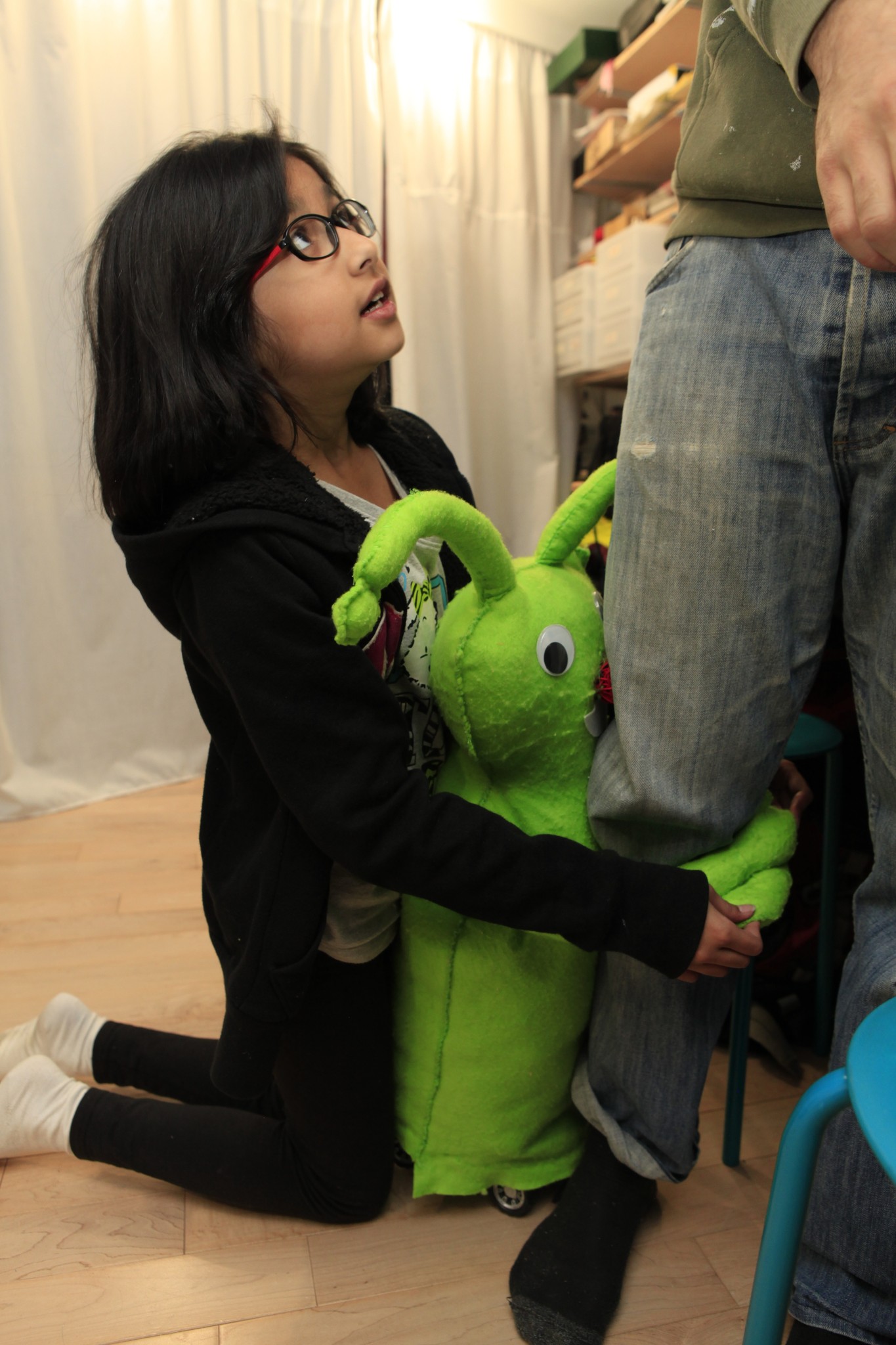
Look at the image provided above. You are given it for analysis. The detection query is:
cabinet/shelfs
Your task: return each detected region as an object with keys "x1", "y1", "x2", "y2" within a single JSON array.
[{"x1": 522, "y1": 0, "x2": 704, "y2": 387}]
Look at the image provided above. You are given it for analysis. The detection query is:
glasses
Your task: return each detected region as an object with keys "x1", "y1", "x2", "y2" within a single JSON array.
[{"x1": 247, "y1": 199, "x2": 376, "y2": 289}]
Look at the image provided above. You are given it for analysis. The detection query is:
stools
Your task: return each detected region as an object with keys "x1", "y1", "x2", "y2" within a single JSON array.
[
  {"x1": 742, "y1": 995, "x2": 896, "y2": 1345},
  {"x1": 721, "y1": 715, "x2": 844, "y2": 1167}
]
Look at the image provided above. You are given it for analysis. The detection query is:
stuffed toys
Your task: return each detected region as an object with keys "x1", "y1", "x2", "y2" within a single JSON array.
[{"x1": 328, "y1": 440, "x2": 798, "y2": 1211}]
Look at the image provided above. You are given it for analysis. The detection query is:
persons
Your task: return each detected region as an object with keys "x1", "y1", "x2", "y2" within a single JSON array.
[
  {"x1": 0, "y1": 122, "x2": 763, "y2": 1229},
  {"x1": 503, "y1": 2, "x2": 896, "y2": 1345}
]
